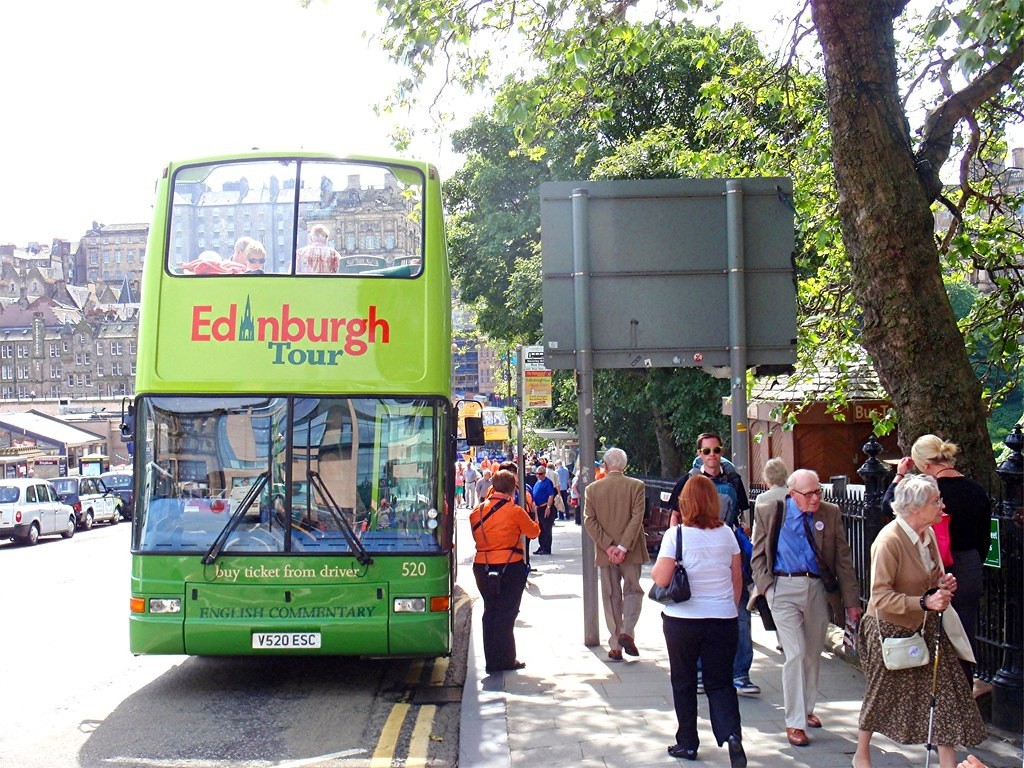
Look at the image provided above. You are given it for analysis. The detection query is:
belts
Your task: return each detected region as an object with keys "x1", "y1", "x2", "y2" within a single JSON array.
[
  {"x1": 775, "y1": 572, "x2": 822, "y2": 580},
  {"x1": 466, "y1": 480, "x2": 476, "y2": 483}
]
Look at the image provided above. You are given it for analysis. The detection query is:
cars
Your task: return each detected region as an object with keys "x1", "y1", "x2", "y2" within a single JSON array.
[
  {"x1": 47, "y1": 477, "x2": 124, "y2": 531},
  {"x1": 98, "y1": 470, "x2": 133, "y2": 521},
  {"x1": 0, "y1": 478, "x2": 76, "y2": 546}
]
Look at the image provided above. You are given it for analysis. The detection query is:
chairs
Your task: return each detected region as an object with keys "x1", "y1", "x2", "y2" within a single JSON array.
[{"x1": 337, "y1": 254, "x2": 423, "y2": 278}]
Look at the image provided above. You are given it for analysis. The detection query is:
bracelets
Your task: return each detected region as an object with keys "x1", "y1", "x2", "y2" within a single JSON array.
[{"x1": 547, "y1": 504, "x2": 551, "y2": 508}]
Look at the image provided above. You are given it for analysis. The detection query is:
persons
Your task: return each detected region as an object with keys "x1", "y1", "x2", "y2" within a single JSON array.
[
  {"x1": 851, "y1": 434, "x2": 991, "y2": 768},
  {"x1": 469, "y1": 470, "x2": 541, "y2": 670},
  {"x1": 650, "y1": 475, "x2": 747, "y2": 768},
  {"x1": 289, "y1": 224, "x2": 341, "y2": 273},
  {"x1": 485, "y1": 461, "x2": 537, "y2": 571},
  {"x1": 669, "y1": 432, "x2": 761, "y2": 694},
  {"x1": 751, "y1": 469, "x2": 863, "y2": 745},
  {"x1": 583, "y1": 447, "x2": 649, "y2": 660},
  {"x1": 751, "y1": 456, "x2": 789, "y2": 652},
  {"x1": 223, "y1": 237, "x2": 266, "y2": 270},
  {"x1": 378, "y1": 496, "x2": 402, "y2": 529},
  {"x1": 456, "y1": 448, "x2": 582, "y2": 555},
  {"x1": 293, "y1": 483, "x2": 313, "y2": 501}
]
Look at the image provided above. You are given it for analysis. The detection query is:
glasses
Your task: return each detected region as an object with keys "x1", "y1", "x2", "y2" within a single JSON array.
[
  {"x1": 535, "y1": 472, "x2": 545, "y2": 476},
  {"x1": 699, "y1": 446, "x2": 726, "y2": 454},
  {"x1": 248, "y1": 257, "x2": 267, "y2": 264},
  {"x1": 793, "y1": 486, "x2": 825, "y2": 498}
]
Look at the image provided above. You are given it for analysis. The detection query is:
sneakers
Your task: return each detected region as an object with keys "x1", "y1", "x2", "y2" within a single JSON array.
[
  {"x1": 734, "y1": 679, "x2": 761, "y2": 694},
  {"x1": 697, "y1": 677, "x2": 706, "y2": 694}
]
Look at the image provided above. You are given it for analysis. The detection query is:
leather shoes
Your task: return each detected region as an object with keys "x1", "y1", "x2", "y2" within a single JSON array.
[
  {"x1": 787, "y1": 727, "x2": 809, "y2": 745},
  {"x1": 806, "y1": 713, "x2": 822, "y2": 728}
]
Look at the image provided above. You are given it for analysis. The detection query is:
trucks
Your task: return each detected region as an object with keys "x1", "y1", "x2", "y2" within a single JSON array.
[{"x1": 229, "y1": 486, "x2": 265, "y2": 522}]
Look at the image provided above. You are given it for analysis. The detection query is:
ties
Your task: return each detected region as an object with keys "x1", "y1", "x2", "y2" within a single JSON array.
[{"x1": 801, "y1": 513, "x2": 839, "y2": 595}]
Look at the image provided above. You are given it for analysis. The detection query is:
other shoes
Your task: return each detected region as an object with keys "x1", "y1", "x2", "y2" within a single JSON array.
[
  {"x1": 608, "y1": 649, "x2": 623, "y2": 660},
  {"x1": 533, "y1": 548, "x2": 552, "y2": 555},
  {"x1": 618, "y1": 633, "x2": 639, "y2": 656},
  {"x1": 728, "y1": 735, "x2": 747, "y2": 768},
  {"x1": 667, "y1": 744, "x2": 698, "y2": 760},
  {"x1": 851, "y1": 753, "x2": 857, "y2": 768},
  {"x1": 457, "y1": 504, "x2": 462, "y2": 508},
  {"x1": 486, "y1": 659, "x2": 525, "y2": 671},
  {"x1": 466, "y1": 505, "x2": 474, "y2": 510}
]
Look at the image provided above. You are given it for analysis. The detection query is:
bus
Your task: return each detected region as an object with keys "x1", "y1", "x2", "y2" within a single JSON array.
[{"x1": 119, "y1": 150, "x2": 484, "y2": 660}]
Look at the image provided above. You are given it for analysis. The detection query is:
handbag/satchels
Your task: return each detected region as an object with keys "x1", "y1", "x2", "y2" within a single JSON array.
[
  {"x1": 931, "y1": 513, "x2": 955, "y2": 568},
  {"x1": 648, "y1": 525, "x2": 691, "y2": 605},
  {"x1": 882, "y1": 632, "x2": 931, "y2": 671}
]
